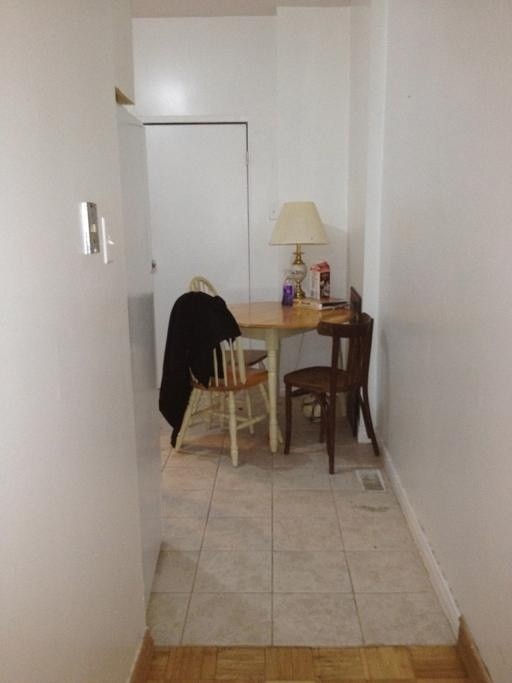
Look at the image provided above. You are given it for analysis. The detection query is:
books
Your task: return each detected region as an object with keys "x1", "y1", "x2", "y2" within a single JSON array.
[{"x1": 293, "y1": 296, "x2": 348, "y2": 310}]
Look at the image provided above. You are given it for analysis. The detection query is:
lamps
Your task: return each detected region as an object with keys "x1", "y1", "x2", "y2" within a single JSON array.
[{"x1": 265, "y1": 198, "x2": 332, "y2": 304}]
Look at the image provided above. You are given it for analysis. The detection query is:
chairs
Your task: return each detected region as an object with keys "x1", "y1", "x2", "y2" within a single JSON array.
[
  {"x1": 188, "y1": 275, "x2": 284, "y2": 437},
  {"x1": 278, "y1": 310, "x2": 381, "y2": 477},
  {"x1": 168, "y1": 292, "x2": 284, "y2": 468}
]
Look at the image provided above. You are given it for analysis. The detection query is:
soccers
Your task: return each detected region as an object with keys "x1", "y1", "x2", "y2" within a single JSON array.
[{"x1": 301, "y1": 394, "x2": 322, "y2": 424}]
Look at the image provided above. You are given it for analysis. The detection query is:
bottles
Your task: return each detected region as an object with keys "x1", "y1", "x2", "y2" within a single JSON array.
[{"x1": 281, "y1": 273, "x2": 294, "y2": 307}]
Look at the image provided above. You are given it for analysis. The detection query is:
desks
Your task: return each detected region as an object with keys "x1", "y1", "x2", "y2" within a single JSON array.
[{"x1": 211, "y1": 296, "x2": 360, "y2": 457}]
